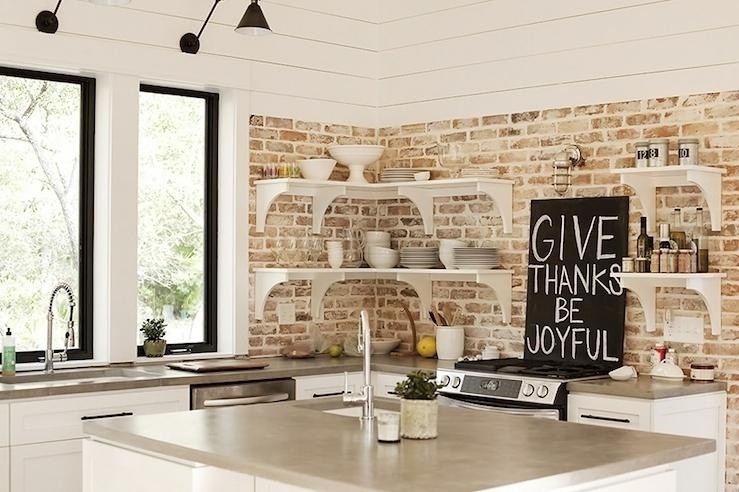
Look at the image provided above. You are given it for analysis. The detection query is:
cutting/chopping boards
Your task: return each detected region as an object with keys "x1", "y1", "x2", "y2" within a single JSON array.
[{"x1": 164, "y1": 358, "x2": 272, "y2": 374}]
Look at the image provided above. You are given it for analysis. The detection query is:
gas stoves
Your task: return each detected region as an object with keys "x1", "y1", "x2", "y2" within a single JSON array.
[{"x1": 435, "y1": 353, "x2": 625, "y2": 409}]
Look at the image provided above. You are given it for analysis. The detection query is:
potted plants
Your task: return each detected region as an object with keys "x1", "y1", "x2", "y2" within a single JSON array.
[
  {"x1": 392, "y1": 367, "x2": 445, "y2": 441},
  {"x1": 139, "y1": 317, "x2": 169, "y2": 359}
]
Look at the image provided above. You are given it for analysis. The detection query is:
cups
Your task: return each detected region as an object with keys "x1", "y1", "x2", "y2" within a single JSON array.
[
  {"x1": 261, "y1": 162, "x2": 301, "y2": 178},
  {"x1": 375, "y1": 409, "x2": 403, "y2": 446},
  {"x1": 436, "y1": 323, "x2": 466, "y2": 361}
]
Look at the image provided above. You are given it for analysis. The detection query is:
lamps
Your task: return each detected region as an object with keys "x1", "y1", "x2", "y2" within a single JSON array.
[
  {"x1": 177, "y1": 0, "x2": 274, "y2": 53},
  {"x1": 551, "y1": 143, "x2": 583, "y2": 197}
]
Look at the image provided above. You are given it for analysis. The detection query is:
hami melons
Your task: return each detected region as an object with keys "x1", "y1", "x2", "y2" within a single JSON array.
[{"x1": 416, "y1": 336, "x2": 436, "y2": 358}]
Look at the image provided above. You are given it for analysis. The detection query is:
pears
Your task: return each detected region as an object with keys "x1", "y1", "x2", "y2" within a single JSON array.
[{"x1": 329, "y1": 345, "x2": 343, "y2": 358}]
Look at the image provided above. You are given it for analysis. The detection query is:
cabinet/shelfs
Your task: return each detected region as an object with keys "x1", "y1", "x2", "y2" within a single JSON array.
[
  {"x1": 609, "y1": 162, "x2": 728, "y2": 337},
  {"x1": 564, "y1": 386, "x2": 729, "y2": 492},
  {"x1": 251, "y1": 176, "x2": 515, "y2": 325},
  {"x1": 2, "y1": 392, "x2": 11, "y2": 492},
  {"x1": 11, "y1": 380, "x2": 193, "y2": 492},
  {"x1": 367, "y1": 363, "x2": 438, "y2": 401},
  {"x1": 295, "y1": 363, "x2": 367, "y2": 401}
]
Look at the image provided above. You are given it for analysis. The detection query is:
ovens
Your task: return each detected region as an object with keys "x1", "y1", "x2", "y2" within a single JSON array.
[
  {"x1": 434, "y1": 395, "x2": 567, "y2": 422},
  {"x1": 191, "y1": 377, "x2": 298, "y2": 413}
]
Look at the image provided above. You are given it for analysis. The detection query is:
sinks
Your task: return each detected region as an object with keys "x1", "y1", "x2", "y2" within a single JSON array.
[
  {"x1": 295, "y1": 395, "x2": 403, "y2": 421},
  {"x1": 1, "y1": 367, "x2": 162, "y2": 384}
]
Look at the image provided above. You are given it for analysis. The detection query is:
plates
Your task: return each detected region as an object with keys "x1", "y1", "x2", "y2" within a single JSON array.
[
  {"x1": 380, "y1": 167, "x2": 432, "y2": 182},
  {"x1": 461, "y1": 169, "x2": 499, "y2": 179},
  {"x1": 281, "y1": 345, "x2": 311, "y2": 359},
  {"x1": 399, "y1": 243, "x2": 438, "y2": 270},
  {"x1": 452, "y1": 246, "x2": 501, "y2": 270}
]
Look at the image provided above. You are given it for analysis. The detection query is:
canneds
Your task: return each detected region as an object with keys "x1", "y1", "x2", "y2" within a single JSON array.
[
  {"x1": 622, "y1": 256, "x2": 635, "y2": 273},
  {"x1": 645, "y1": 259, "x2": 650, "y2": 272},
  {"x1": 634, "y1": 257, "x2": 647, "y2": 273}
]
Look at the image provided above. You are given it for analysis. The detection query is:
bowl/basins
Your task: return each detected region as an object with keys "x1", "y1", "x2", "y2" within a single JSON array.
[
  {"x1": 369, "y1": 337, "x2": 402, "y2": 355},
  {"x1": 295, "y1": 159, "x2": 337, "y2": 181},
  {"x1": 438, "y1": 235, "x2": 467, "y2": 270},
  {"x1": 363, "y1": 231, "x2": 399, "y2": 270},
  {"x1": 326, "y1": 241, "x2": 345, "y2": 269}
]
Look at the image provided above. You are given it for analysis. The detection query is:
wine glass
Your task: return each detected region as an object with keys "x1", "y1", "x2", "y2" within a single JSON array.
[
  {"x1": 326, "y1": 144, "x2": 387, "y2": 185},
  {"x1": 438, "y1": 144, "x2": 470, "y2": 179}
]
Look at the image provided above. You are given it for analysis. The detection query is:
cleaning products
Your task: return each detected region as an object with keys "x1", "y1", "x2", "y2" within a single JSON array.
[{"x1": 2, "y1": 328, "x2": 16, "y2": 375}]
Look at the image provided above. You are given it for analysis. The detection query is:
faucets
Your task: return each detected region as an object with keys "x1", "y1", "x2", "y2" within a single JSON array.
[
  {"x1": 357, "y1": 307, "x2": 372, "y2": 382},
  {"x1": 49, "y1": 282, "x2": 76, "y2": 348}
]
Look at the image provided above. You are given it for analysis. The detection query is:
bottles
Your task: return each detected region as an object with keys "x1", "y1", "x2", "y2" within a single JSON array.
[
  {"x1": 481, "y1": 344, "x2": 500, "y2": 361},
  {"x1": 634, "y1": 138, "x2": 699, "y2": 167},
  {"x1": 621, "y1": 208, "x2": 708, "y2": 273},
  {"x1": 1, "y1": 327, "x2": 17, "y2": 378},
  {"x1": 648, "y1": 341, "x2": 687, "y2": 370}
]
